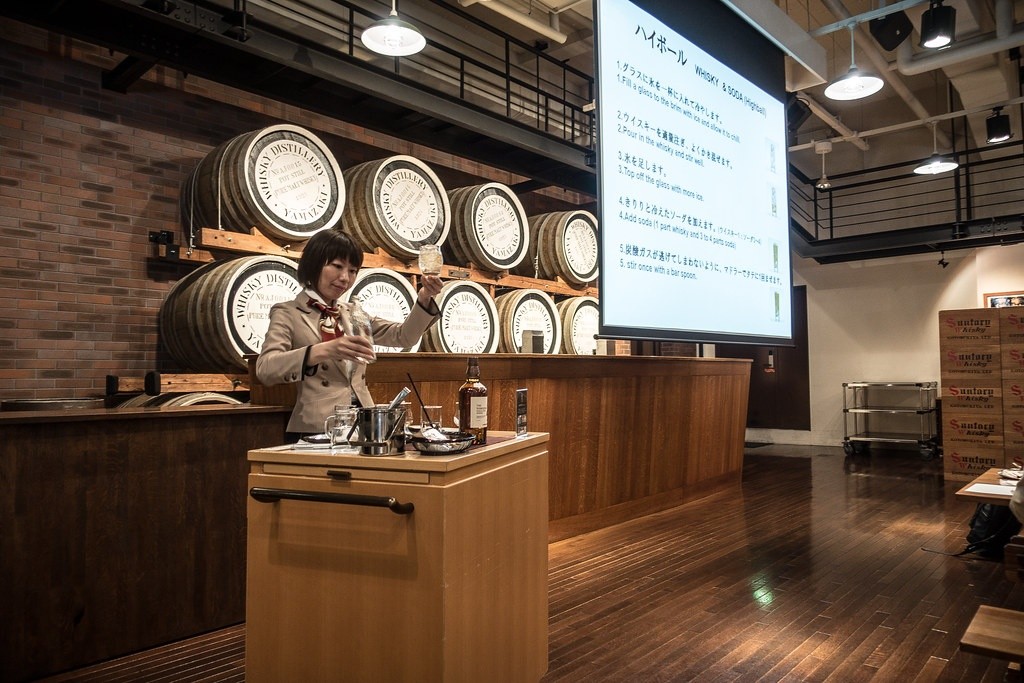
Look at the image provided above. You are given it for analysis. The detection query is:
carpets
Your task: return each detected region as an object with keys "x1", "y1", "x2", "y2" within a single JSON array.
[{"x1": 744, "y1": 441, "x2": 774, "y2": 449}]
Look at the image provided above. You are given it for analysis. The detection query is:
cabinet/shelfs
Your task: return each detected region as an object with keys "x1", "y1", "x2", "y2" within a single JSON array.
[{"x1": 841, "y1": 380, "x2": 939, "y2": 461}]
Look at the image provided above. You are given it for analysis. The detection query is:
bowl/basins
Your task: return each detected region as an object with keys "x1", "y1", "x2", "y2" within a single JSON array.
[{"x1": 346, "y1": 406, "x2": 407, "y2": 457}]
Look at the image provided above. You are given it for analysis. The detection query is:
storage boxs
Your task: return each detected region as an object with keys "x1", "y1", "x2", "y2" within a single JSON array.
[{"x1": 938, "y1": 306, "x2": 1024, "y2": 481}]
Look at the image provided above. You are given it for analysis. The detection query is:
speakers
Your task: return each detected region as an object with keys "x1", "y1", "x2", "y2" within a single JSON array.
[{"x1": 870, "y1": 10, "x2": 913, "y2": 51}]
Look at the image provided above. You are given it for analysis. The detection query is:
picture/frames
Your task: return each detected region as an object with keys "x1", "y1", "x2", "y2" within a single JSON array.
[{"x1": 983, "y1": 291, "x2": 1024, "y2": 308}]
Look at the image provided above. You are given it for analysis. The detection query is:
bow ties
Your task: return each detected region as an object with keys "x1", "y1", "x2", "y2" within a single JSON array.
[{"x1": 307, "y1": 297, "x2": 345, "y2": 343}]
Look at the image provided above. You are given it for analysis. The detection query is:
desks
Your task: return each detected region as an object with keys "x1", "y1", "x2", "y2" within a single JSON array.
[
  {"x1": 0, "y1": 403, "x2": 296, "y2": 683},
  {"x1": 954, "y1": 466, "x2": 1024, "y2": 506},
  {"x1": 243, "y1": 425, "x2": 549, "y2": 683}
]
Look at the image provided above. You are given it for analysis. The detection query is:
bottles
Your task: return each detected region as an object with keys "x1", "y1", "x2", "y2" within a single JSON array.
[
  {"x1": 349, "y1": 295, "x2": 377, "y2": 364},
  {"x1": 459, "y1": 357, "x2": 488, "y2": 445},
  {"x1": 518, "y1": 393, "x2": 526, "y2": 436}
]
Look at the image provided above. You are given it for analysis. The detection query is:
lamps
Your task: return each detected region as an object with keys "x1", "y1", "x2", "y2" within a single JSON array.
[
  {"x1": 360, "y1": 0, "x2": 427, "y2": 57},
  {"x1": 824, "y1": 21, "x2": 885, "y2": 101},
  {"x1": 912, "y1": 119, "x2": 959, "y2": 175},
  {"x1": 984, "y1": 105, "x2": 1015, "y2": 145},
  {"x1": 937, "y1": 258, "x2": 949, "y2": 269},
  {"x1": 917, "y1": 0, "x2": 958, "y2": 50},
  {"x1": 814, "y1": 141, "x2": 833, "y2": 189}
]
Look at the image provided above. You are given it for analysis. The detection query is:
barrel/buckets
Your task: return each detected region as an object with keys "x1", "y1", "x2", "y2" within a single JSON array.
[
  {"x1": 337, "y1": 265, "x2": 422, "y2": 354},
  {"x1": 182, "y1": 124, "x2": 345, "y2": 245},
  {"x1": 158, "y1": 255, "x2": 307, "y2": 377},
  {"x1": 527, "y1": 211, "x2": 601, "y2": 287},
  {"x1": 114, "y1": 392, "x2": 246, "y2": 409},
  {"x1": 420, "y1": 280, "x2": 500, "y2": 354},
  {"x1": 496, "y1": 290, "x2": 563, "y2": 354},
  {"x1": 555, "y1": 296, "x2": 601, "y2": 355},
  {"x1": 332, "y1": 155, "x2": 451, "y2": 262},
  {"x1": 443, "y1": 182, "x2": 530, "y2": 273}
]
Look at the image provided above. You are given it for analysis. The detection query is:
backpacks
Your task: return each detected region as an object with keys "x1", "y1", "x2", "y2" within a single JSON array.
[{"x1": 961, "y1": 501, "x2": 1024, "y2": 556}]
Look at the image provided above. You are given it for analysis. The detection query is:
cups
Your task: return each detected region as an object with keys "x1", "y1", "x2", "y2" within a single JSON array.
[
  {"x1": 419, "y1": 244, "x2": 443, "y2": 278},
  {"x1": 325, "y1": 404, "x2": 361, "y2": 455},
  {"x1": 421, "y1": 405, "x2": 442, "y2": 430}
]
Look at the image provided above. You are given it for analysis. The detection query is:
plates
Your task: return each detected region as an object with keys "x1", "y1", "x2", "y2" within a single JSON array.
[
  {"x1": 409, "y1": 424, "x2": 420, "y2": 433},
  {"x1": 998, "y1": 470, "x2": 1024, "y2": 480},
  {"x1": 408, "y1": 431, "x2": 476, "y2": 452}
]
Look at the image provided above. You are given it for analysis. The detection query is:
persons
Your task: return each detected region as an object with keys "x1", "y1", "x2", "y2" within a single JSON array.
[
  {"x1": 1009, "y1": 471, "x2": 1024, "y2": 522},
  {"x1": 256, "y1": 230, "x2": 444, "y2": 442}
]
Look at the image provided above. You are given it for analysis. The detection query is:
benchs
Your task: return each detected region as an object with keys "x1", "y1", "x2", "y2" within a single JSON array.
[{"x1": 959, "y1": 604, "x2": 1024, "y2": 683}]
